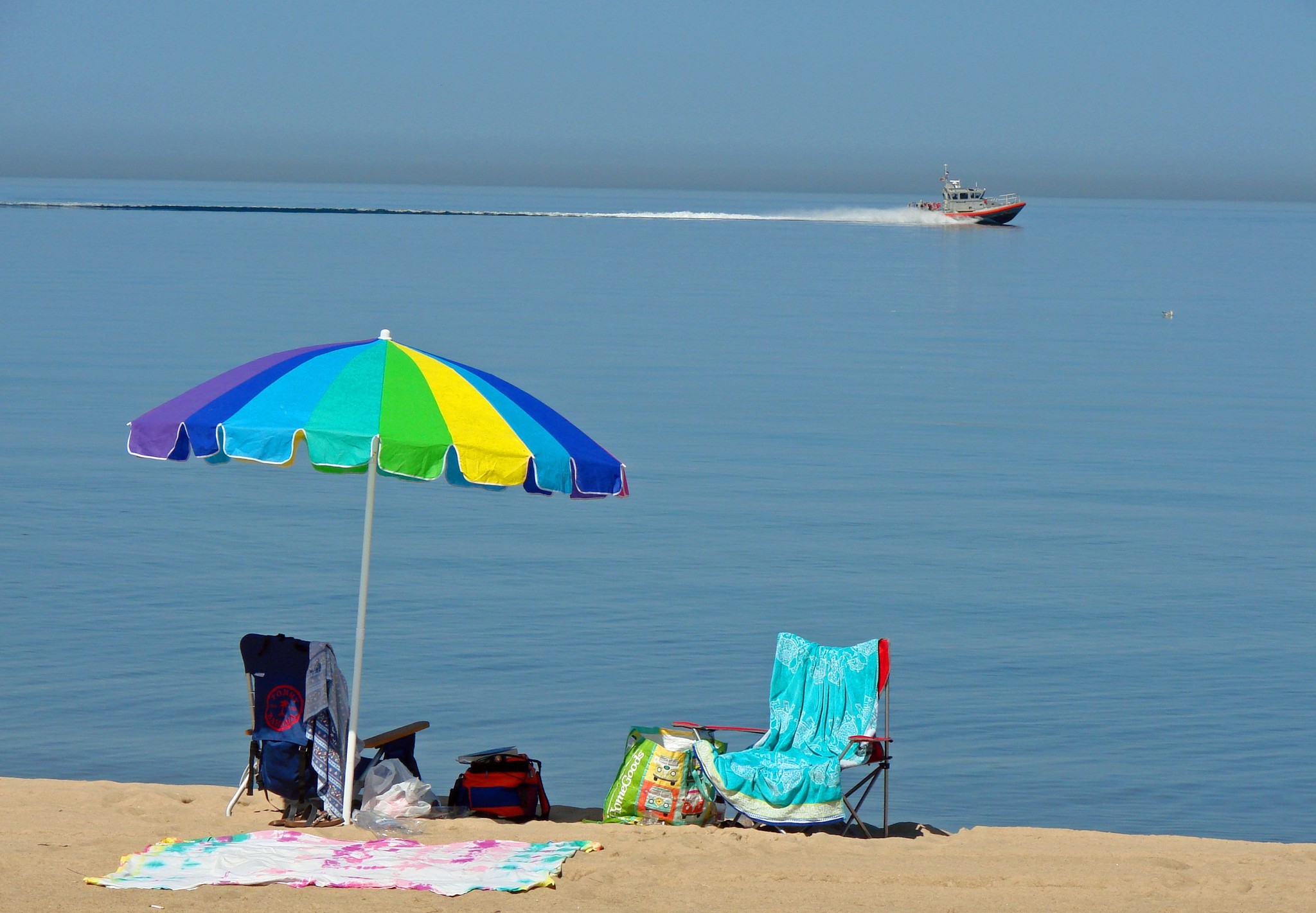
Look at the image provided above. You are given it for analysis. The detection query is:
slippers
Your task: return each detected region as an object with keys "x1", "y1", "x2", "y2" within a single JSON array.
[
  {"x1": 286, "y1": 804, "x2": 345, "y2": 828},
  {"x1": 268, "y1": 803, "x2": 301, "y2": 826}
]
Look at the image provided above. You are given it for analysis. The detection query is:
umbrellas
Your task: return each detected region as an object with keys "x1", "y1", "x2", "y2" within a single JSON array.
[{"x1": 127, "y1": 329, "x2": 628, "y2": 824}]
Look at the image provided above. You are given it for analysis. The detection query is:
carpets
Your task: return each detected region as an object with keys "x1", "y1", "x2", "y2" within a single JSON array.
[{"x1": 76, "y1": 829, "x2": 605, "y2": 892}]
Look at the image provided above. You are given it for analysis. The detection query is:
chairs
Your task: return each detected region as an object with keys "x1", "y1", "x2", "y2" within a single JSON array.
[
  {"x1": 224, "y1": 631, "x2": 433, "y2": 829},
  {"x1": 670, "y1": 630, "x2": 895, "y2": 842}
]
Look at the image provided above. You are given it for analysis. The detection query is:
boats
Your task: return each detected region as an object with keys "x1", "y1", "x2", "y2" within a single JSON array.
[{"x1": 908, "y1": 164, "x2": 1026, "y2": 226}]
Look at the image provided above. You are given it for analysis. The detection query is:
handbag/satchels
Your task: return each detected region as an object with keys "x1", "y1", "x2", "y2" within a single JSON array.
[
  {"x1": 463, "y1": 753, "x2": 550, "y2": 821},
  {"x1": 604, "y1": 726, "x2": 728, "y2": 826},
  {"x1": 360, "y1": 757, "x2": 442, "y2": 819}
]
